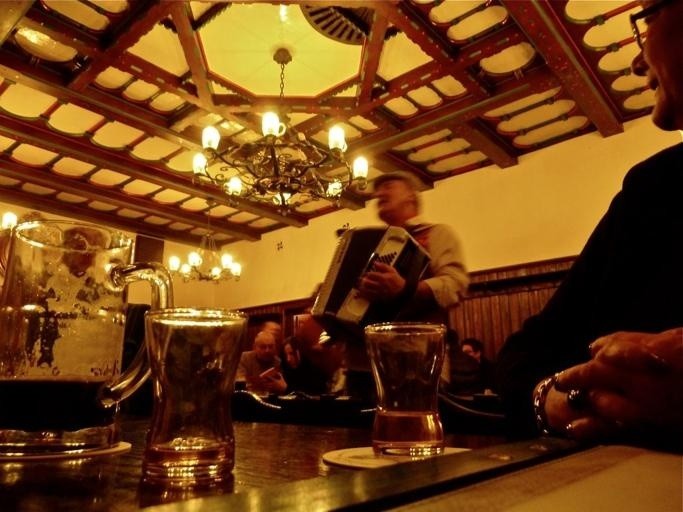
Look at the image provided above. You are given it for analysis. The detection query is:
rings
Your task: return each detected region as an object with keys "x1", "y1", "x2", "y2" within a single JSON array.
[{"x1": 568, "y1": 388, "x2": 585, "y2": 410}]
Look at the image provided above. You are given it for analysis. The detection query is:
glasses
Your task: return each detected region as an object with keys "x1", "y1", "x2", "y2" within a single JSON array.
[{"x1": 629, "y1": 1, "x2": 678, "y2": 49}]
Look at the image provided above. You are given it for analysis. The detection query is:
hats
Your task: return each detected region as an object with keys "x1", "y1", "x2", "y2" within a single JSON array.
[{"x1": 374, "y1": 170, "x2": 416, "y2": 187}]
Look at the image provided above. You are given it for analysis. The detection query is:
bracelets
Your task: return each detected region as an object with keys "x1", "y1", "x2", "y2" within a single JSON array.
[{"x1": 534, "y1": 376, "x2": 556, "y2": 437}]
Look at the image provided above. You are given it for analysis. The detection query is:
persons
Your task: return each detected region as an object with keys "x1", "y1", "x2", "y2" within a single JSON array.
[
  {"x1": 235, "y1": 322, "x2": 496, "y2": 397},
  {"x1": 497, "y1": 1, "x2": 682, "y2": 454},
  {"x1": 309, "y1": 170, "x2": 468, "y2": 432}
]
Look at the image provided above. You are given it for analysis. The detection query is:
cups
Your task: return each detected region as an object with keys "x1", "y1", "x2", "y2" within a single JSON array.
[
  {"x1": 0, "y1": 221, "x2": 174, "y2": 465},
  {"x1": 364, "y1": 322, "x2": 448, "y2": 455},
  {"x1": 139, "y1": 305, "x2": 249, "y2": 484}
]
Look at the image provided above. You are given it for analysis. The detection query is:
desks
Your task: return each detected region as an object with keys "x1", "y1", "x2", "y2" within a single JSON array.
[{"x1": 2, "y1": 389, "x2": 683, "y2": 512}]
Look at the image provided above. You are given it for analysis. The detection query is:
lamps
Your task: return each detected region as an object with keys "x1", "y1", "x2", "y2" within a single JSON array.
[
  {"x1": 160, "y1": 200, "x2": 241, "y2": 284},
  {"x1": 187, "y1": 47, "x2": 370, "y2": 214}
]
